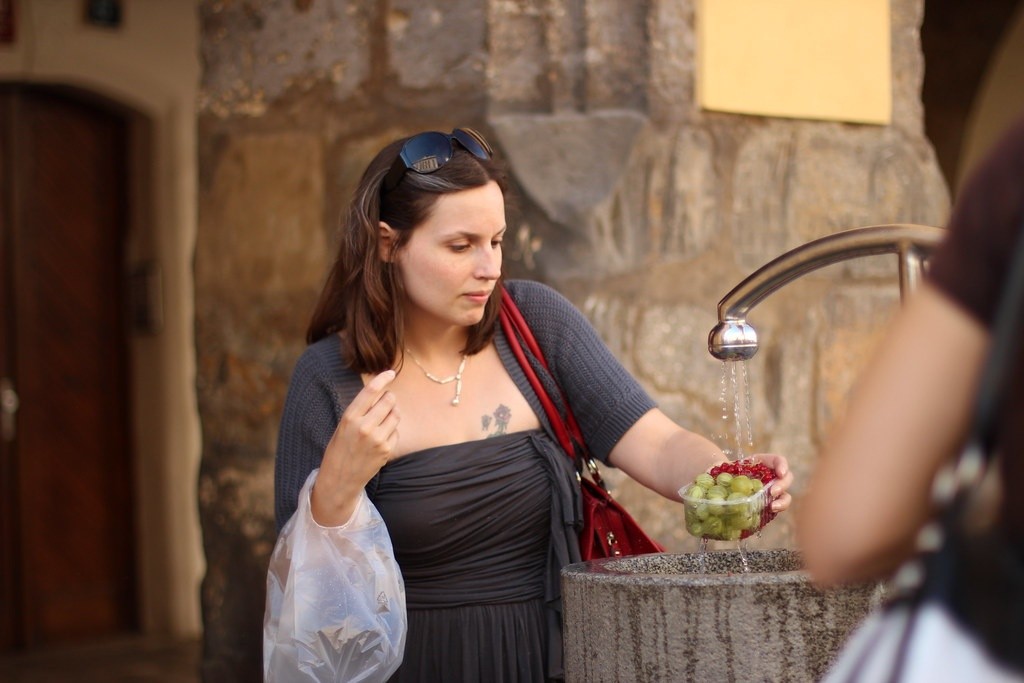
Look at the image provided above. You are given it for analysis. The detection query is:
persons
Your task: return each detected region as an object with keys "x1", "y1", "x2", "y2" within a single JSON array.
[
  {"x1": 276, "y1": 132, "x2": 793, "y2": 683},
  {"x1": 799, "y1": 19, "x2": 1023, "y2": 683}
]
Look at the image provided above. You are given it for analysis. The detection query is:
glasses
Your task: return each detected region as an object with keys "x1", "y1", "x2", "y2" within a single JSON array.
[{"x1": 380, "y1": 126, "x2": 494, "y2": 206}]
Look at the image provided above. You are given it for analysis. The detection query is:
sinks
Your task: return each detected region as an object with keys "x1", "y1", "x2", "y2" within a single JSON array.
[{"x1": 560, "y1": 549, "x2": 888, "y2": 683}]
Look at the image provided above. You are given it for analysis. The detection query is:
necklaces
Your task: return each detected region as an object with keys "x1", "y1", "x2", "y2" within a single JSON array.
[{"x1": 405, "y1": 347, "x2": 468, "y2": 405}]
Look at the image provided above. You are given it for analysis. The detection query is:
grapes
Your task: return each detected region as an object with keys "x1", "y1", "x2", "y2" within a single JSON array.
[{"x1": 683, "y1": 472, "x2": 767, "y2": 541}]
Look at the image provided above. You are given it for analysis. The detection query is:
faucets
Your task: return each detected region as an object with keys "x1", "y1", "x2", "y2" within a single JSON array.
[{"x1": 706, "y1": 222, "x2": 952, "y2": 362}]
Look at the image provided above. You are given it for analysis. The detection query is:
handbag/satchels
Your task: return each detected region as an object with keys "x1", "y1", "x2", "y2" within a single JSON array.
[
  {"x1": 819, "y1": 562, "x2": 1016, "y2": 683},
  {"x1": 262, "y1": 468, "x2": 407, "y2": 683},
  {"x1": 497, "y1": 282, "x2": 668, "y2": 562}
]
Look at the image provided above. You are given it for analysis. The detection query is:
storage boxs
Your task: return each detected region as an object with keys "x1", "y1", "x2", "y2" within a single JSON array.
[{"x1": 677, "y1": 475, "x2": 777, "y2": 541}]
